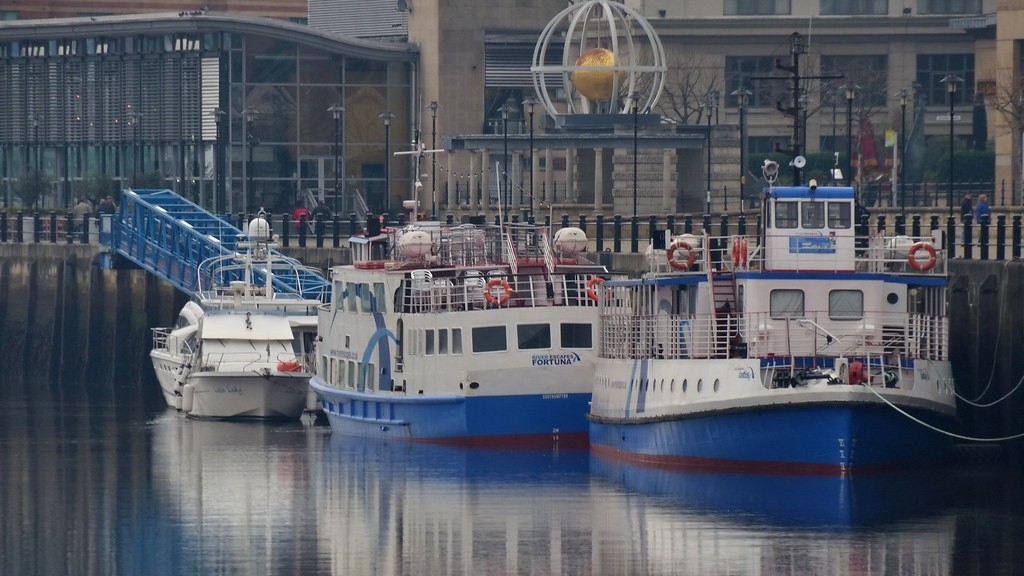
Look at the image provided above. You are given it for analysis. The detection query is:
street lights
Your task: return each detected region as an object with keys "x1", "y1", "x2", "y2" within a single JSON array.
[
  {"x1": 424, "y1": 100, "x2": 442, "y2": 220},
  {"x1": 939, "y1": 74, "x2": 965, "y2": 216},
  {"x1": 520, "y1": 97, "x2": 540, "y2": 223},
  {"x1": 30, "y1": 115, "x2": 43, "y2": 217},
  {"x1": 327, "y1": 104, "x2": 346, "y2": 215},
  {"x1": 730, "y1": 86, "x2": 752, "y2": 218},
  {"x1": 126, "y1": 111, "x2": 144, "y2": 190},
  {"x1": 497, "y1": 104, "x2": 515, "y2": 221},
  {"x1": 379, "y1": 111, "x2": 396, "y2": 212},
  {"x1": 701, "y1": 102, "x2": 721, "y2": 214},
  {"x1": 626, "y1": 90, "x2": 647, "y2": 216},
  {"x1": 209, "y1": 108, "x2": 230, "y2": 215},
  {"x1": 838, "y1": 82, "x2": 862, "y2": 186},
  {"x1": 893, "y1": 89, "x2": 914, "y2": 215},
  {"x1": 241, "y1": 107, "x2": 261, "y2": 217}
]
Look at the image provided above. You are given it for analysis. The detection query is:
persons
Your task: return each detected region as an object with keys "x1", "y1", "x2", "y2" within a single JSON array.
[
  {"x1": 106, "y1": 195, "x2": 116, "y2": 214},
  {"x1": 294, "y1": 202, "x2": 310, "y2": 238},
  {"x1": 72, "y1": 199, "x2": 92, "y2": 243},
  {"x1": 976, "y1": 194, "x2": 991, "y2": 247},
  {"x1": 855, "y1": 196, "x2": 870, "y2": 256},
  {"x1": 309, "y1": 200, "x2": 332, "y2": 244},
  {"x1": 95, "y1": 199, "x2": 114, "y2": 225},
  {"x1": 960, "y1": 192, "x2": 974, "y2": 247}
]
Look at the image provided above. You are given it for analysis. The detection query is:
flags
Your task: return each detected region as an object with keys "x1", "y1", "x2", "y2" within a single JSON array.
[
  {"x1": 908, "y1": 109, "x2": 927, "y2": 164},
  {"x1": 861, "y1": 111, "x2": 880, "y2": 165}
]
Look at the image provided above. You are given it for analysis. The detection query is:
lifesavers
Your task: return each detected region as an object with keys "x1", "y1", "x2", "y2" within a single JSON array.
[
  {"x1": 733, "y1": 239, "x2": 740, "y2": 266},
  {"x1": 908, "y1": 242, "x2": 936, "y2": 271},
  {"x1": 484, "y1": 279, "x2": 511, "y2": 304},
  {"x1": 587, "y1": 277, "x2": 611, "y2": 302},
  {"x1": 667, "y1": 242, "x2": 696, "y2": 269},
  {"x1": 740, "y1": 239, "x2": 747, "y2": 267}
]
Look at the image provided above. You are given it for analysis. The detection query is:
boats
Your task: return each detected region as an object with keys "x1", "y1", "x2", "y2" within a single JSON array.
[
  {"x1": 307, "y1": 131, "x2": 633, "y2": 450},
  {"x1": 149, "y1": 213, "x2": 312, "y2": 423},
  {"x1": 586, "y1": 155, "x2": 964, "y2": 479}
]
